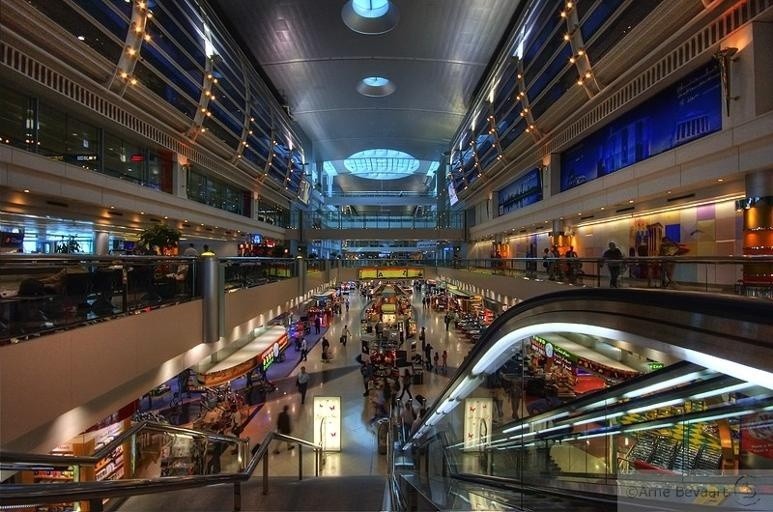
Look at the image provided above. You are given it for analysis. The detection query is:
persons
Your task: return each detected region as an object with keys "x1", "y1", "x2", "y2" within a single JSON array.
[
  {"x1": 356, "y1": 271, "x2": 452, "y2": 435},
  {"x1": 343, "y1": 297, "x2": 349, "y2": 312},
  {"x1": 565, "y1": 244, "x2": 579, "y2": 283},
  {"x1": 274, "y1": 403, "x2": 296, "y2": 453},
  {"x1": 492, "y1": 383, "x2": 508, "y2": 418},
  {"x1": 507, "y1": 381, "x2": 522, "y2": 421},
  {"x1": 38, "y1": 255, "x2": 91, "y2": 285},
  {"x1": 295, "y1": 364, "x2": 310, "y2": 406},
  {"x1": 166, "y1": 255, "x2": 190, "y2": 284},
  {"x1": 319, "y1": 335, "x2": 331, "y2": 364},
  {"x1": 597, "y1": 239, "x2": 622, "y2": 286},
  {"x1": 526, "y1": 385, "x2": 573, "y2": 440},
  {"x1": 550, "y1": 244, "x2": 564, "y2": 281},
  {"x1": 298, "y1": 336, "x2": 307, "y2": 362},
  {"x1": 339, "y1": 324, "x2": 351, "y2": 346},
  {"x1": 314, "y1": 313, "x2": 321, "y2": 334},
  {"x1": 203, "y1": 244, "x2": 216, "y2": 254},
  {"x1": 657, "y1": 236, "x2": 680, "y2": 289},
  {"x1": 229, "y1": 404, "x2": 241, "y2": 454},
  {"x1": 184, "y1": 242, "x2": 198, "y2": 256},
  {"x1": 542, "y1": 246, "x2": 557, "y2": 281}
]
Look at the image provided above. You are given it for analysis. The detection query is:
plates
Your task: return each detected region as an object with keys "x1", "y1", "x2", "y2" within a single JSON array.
[
  {"x1": 34, "y1": 435, "x2": 125, "y2": 512},
  {"x1": 425, "y1": 280, "x2": 578, "y2": 400}
]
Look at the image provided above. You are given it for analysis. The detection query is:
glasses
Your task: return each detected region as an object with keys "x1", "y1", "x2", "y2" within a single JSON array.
[
  {"x1": 713, "y1": 46, "x2": 740, "y2": 118},
  {"x1": 450, "y1": 1, "x2": 601, "y2": 197},
  {"x1": 116, "y1": 0, "x2": 295, "y2": 192}
]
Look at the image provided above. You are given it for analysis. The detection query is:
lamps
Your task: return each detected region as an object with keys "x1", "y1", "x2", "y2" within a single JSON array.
[{"x1": 6, "y1": 261, "x2": 268, "y2": 330}]
